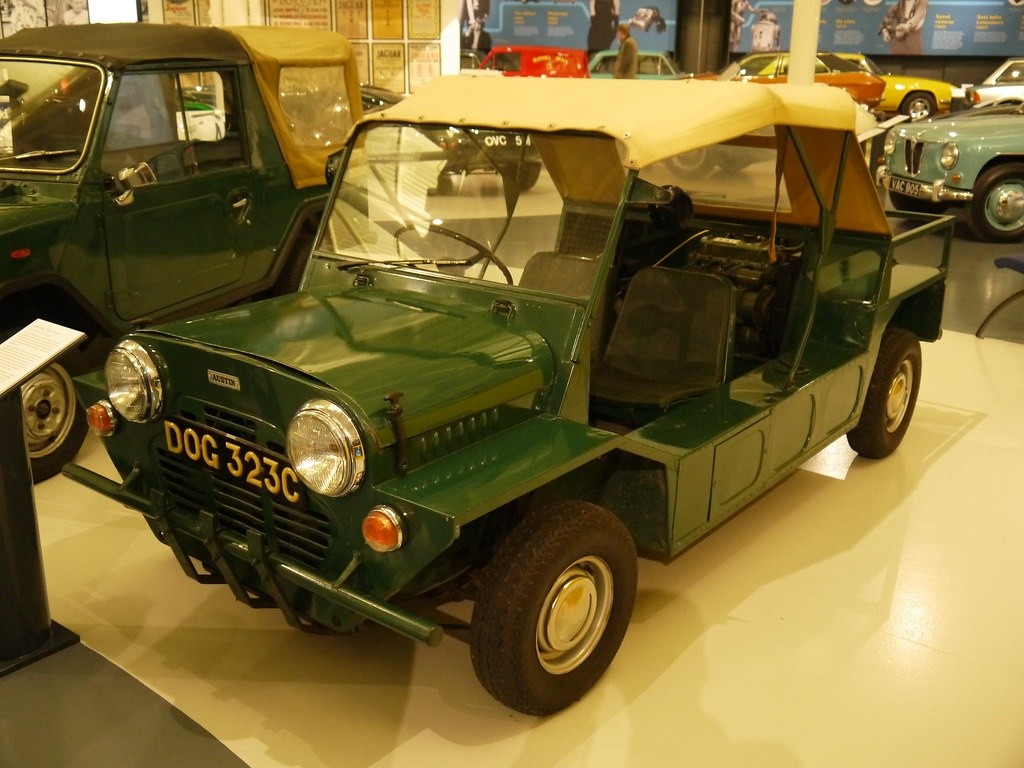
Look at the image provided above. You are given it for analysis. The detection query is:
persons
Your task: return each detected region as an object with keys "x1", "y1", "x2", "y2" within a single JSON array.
[
  {"x1": 64, "y1": 0, "x2": 88, "y2": 25},
  {"x1": 459, "y1": 0, "x2": 491, "y2": 54},
  {"x1": 879, "y1": 0, "x2": 928, "y2": 55},
  {"x1": 728, "y1": 0, "x2": 781, "y2": 53},
  {"x1": 613, "y1": 23, "x2": 637, "y2": 80}
]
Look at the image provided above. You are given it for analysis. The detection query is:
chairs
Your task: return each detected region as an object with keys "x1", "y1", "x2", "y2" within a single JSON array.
[
  {"x1": 591, "y1": 266, "x2": 736, "y2": 428},
  {"x1": 514, "y1": 248, "x2": 607, "y2": 368}
]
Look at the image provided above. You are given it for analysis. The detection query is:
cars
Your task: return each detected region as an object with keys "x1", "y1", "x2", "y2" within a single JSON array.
[
  {"x1": 165, "y1": 46, "x2": 1024, "y2": 197},
  {"x1": 874, "y1": 85, "x2": 1024, "y2": 241}
]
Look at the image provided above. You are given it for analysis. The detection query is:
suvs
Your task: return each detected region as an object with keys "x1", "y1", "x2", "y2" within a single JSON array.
[
  {"x1": 1, "y1": 22, "x2": 372, "y2": 482},
  {"x1": 57, "y1": 74, "x2": 959, "y2": 718}
]
